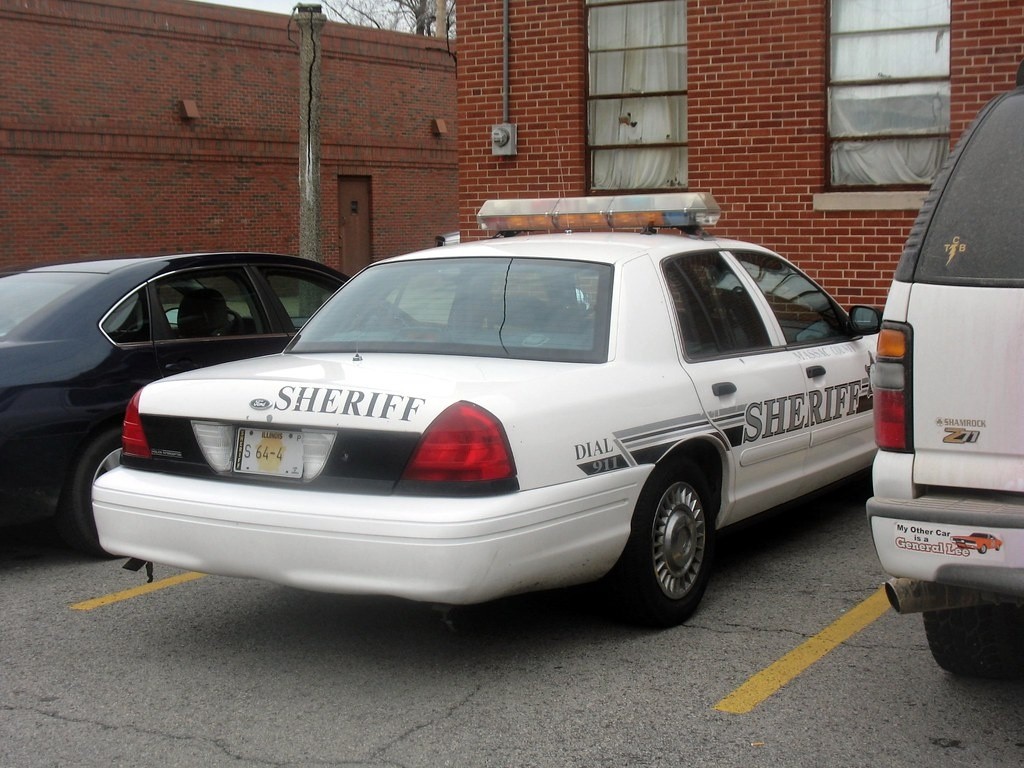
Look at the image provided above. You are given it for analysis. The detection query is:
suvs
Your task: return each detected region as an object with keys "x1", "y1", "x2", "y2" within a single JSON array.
[{"x1": 865, "y1": 87, "x2": 1022, "y2": 677}]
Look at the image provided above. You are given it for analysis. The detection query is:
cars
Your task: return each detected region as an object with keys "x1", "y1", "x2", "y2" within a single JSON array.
[
  {"x1": 91, "y1": 191, "x2": 885, "y2": 626},
  {"x1": 0, "y1": 252, "x2": 356, "y2": 560}
]
